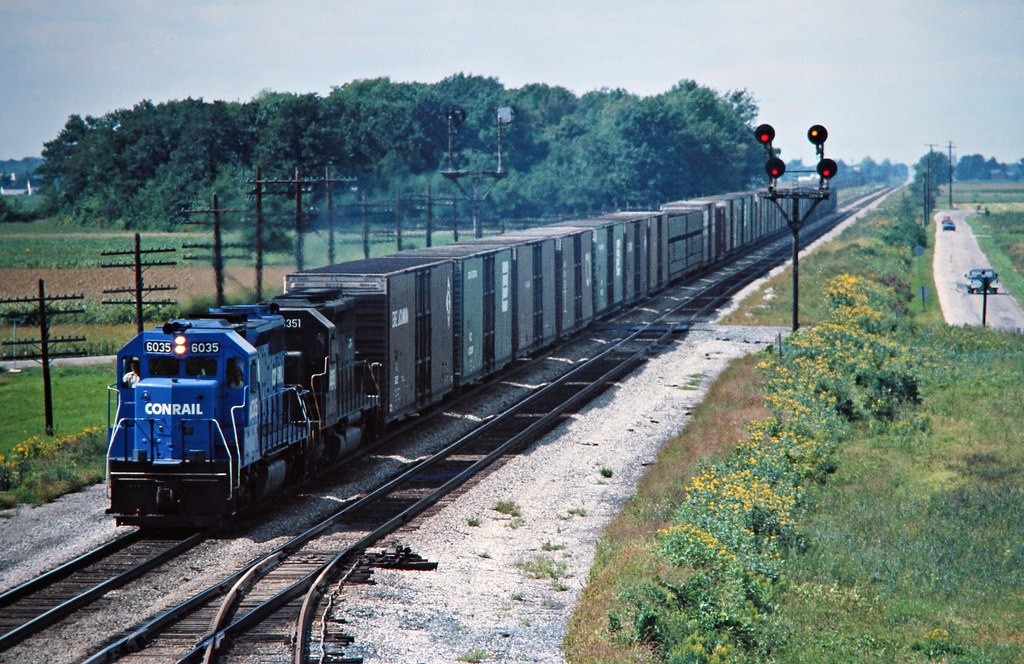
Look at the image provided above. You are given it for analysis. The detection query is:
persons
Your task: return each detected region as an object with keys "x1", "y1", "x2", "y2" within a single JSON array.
[
  {"x1": 230, "y1": 369, "x2": 244, "y2": 388},
  {"x1": 122, "y1": 360, "x2": 140, "y2": 389}
]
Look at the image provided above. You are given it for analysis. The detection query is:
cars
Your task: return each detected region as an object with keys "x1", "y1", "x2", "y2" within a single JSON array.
[
  {"x1": 941, "y1": 215, "x2": 953, "y2": 223},
  {"x1": 965, "y1": 268, "x2": 998, "y2": 295},
  {"x1": 942, "y1": 221, "x2": 955, "y2": 231}
]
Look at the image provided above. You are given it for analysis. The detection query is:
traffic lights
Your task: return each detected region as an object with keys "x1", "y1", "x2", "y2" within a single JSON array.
[
  {"x1": 754, "y1": 124, "x2": 776, "y2": 145},
  {"x1": 766, "y1": 158, "x2": 785, "y2": 178},
  {"x1": 807, "y1": 124, "x2": 828, "y2": 146},
  {"x1": 817, "y1": 159, "x2": 838, "y2": 179}
]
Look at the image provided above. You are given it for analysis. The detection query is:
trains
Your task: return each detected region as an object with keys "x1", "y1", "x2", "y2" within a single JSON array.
[{"x1": 103, "y1": 174, "x2": 837, "y2": 532}]
